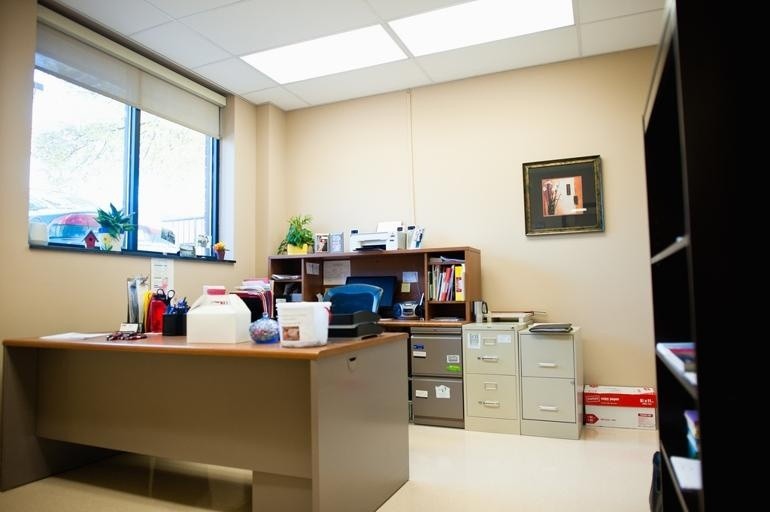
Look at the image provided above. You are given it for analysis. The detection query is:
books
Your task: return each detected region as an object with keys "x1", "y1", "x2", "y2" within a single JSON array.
[
  {"x1": 431, "y1": 316, "x2": 462, "y2": 322},
  {"x1": 125, "y1": 274, "x2": 301, "y2": 336},
  {"x1": 529, "y1": 324, "x2": 572, "y2": 332},
  {"x1": 656, "y1": 339, "x2": 703, "y2": 491},
  {"x1": 430, "y1": 257, "x2": 464, "y2": 301}
]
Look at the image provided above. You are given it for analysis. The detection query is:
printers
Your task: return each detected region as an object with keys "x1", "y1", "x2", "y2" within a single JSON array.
[{"x1": 348, "y1": 225, "x2": 405, "y2": 250}]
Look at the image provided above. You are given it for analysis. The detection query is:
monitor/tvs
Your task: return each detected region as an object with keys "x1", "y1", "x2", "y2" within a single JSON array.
[{"x1": 345, "y1": 276, "x2": 396, "y2": 309}]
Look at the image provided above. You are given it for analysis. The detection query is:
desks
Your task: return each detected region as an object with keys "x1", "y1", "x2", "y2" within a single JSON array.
[{"x1": 1, "y1": 331, "x2": 412, "y2": 512}]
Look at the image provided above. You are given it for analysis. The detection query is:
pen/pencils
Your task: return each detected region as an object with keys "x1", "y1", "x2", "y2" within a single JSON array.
[{"x1": 165, "y1": 297, "x2": 191, "y2": 315}]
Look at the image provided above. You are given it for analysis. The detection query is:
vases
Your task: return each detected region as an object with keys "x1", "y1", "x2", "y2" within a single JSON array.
[{"x1": 215, "y1": 248, "x2": 226, "y2": 261}]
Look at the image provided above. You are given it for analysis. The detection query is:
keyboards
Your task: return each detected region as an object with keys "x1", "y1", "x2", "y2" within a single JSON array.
[{"x1": 378, "y1": 312, "x2": 394, "y2": 318}]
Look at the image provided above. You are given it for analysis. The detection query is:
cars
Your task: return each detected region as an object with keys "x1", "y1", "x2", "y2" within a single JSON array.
[{"x1": 27, "y1": 190, "x2": 116, "y2": 248}]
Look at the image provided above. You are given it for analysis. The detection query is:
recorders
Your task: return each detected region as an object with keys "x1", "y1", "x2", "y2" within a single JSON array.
[{"x1": 393, "y1": 293, "x2": 424, "y2": 318}]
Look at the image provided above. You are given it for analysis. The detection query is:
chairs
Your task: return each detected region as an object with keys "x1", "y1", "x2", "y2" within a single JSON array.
[{"x1": 320, "y1": 284, "x2": 384, "y2": 319}]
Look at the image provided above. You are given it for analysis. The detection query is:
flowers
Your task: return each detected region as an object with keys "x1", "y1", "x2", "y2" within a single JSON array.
[
  {"x1": 212, "y1": 240, "x2": 226, "y2": 249},
  {"x1": 100, "y1": 235, "x2": 113, "y2": 250}
]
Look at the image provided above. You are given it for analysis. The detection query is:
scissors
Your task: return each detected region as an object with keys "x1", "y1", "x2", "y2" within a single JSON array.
[{"x1": 156, "y1": 288, "x2": 175, "y2": 306}]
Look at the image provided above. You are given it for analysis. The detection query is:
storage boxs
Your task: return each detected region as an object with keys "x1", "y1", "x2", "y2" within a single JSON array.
[{"x1": 583, "y1": 384, "x2": 656, "y2": 430}]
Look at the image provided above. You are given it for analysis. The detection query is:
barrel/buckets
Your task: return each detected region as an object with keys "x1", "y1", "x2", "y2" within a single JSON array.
[{"x1": 277, "y1": 301, "x2": 334, "y2": 348}]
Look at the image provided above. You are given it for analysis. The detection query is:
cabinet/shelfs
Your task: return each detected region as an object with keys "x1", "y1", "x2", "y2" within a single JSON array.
[
  {"x1": 462, "y1": 320, "x2": 521, "y2": 433},
  {"x1": 518, "y1": 323, "x2": 587, "y2": 440},
  {"x1": 637, "y1": 1, "x2": 770, "y2": 509},
  {"x1": 409, "y1": 326, "x2": 467, "y2": 429},
  {"x1": 268, "y1": 244, "x2": 482, "y2": 323}
]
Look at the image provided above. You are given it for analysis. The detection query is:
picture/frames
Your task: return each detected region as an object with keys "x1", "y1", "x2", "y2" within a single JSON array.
[
  {"x1": 315, "y1": 234, "x2": 329, "y2": 252},
  {"x1": 329, "y1": 232, "x2": 344, "y2": 252},
  {"x1": 521, "y1": 152, "x2": 607, "y2": 238}
]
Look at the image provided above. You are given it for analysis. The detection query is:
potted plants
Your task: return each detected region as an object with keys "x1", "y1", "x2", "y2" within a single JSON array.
[
  {"x1": 93, "y1": 201, "x2": 137, "y2": 253},
  {"x1": 276, "y1": 215, "x2": 314, "y2": 255}
]
